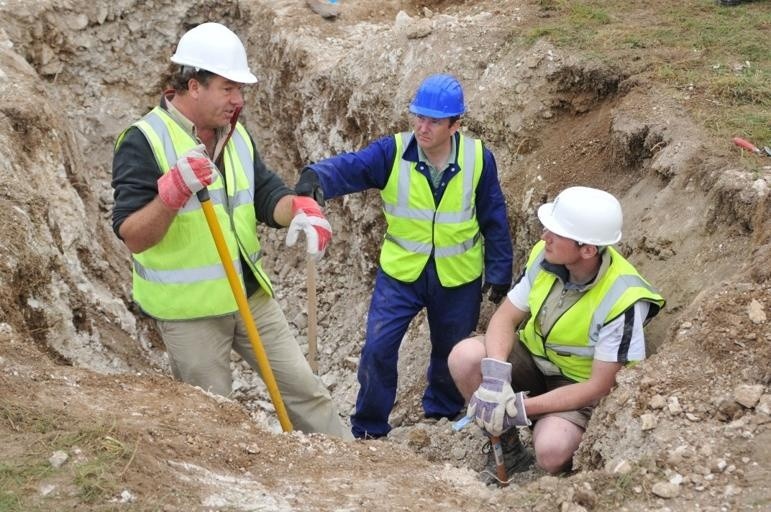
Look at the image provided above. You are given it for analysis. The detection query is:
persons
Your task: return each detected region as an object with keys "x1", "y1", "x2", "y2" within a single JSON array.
[
  {"x1": 111, "y1": 22, "x2": 356, "y2": 442},
  {"x1": 295, "y1": 74, "x2": 513, "y2": 439},
  {"x1": 447, "y1": 186, "x2": 666, "y2": 474}
]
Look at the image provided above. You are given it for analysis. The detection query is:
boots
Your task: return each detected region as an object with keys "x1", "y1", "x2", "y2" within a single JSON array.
[{"x1": 480, "y1": 427, "x2": 534, "y2": 486}]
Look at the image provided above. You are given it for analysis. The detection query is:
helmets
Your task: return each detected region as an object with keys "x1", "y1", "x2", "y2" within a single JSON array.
[
  {"x1": 537, "y1": 186, "x2": 623, "y2": 246},
  {"x1": 409, "y1": 74, "x2": 465, "y2": 118},
  {"x1": 170, "y1": 23, "x2": 258, "y2": 84}
]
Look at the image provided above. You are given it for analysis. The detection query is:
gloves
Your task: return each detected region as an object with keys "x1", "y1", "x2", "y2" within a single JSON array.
[
  {"x1": 468, "y1": 358, "x2": 532, "y2": 437},
  {"x1": 482, "y1": 282, "x2": 510, "y2": 304},
  {"x1": 157, "y1": 144, "x2": 217, "y2": 208},
  {"x1": 286, "y1": 170, "x2": 333, "y2": 261}
]
